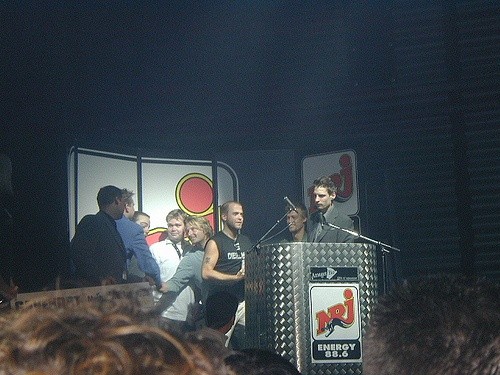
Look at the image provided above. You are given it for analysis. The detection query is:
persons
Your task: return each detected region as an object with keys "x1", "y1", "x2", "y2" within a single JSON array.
[
  {"x1": 2, "y1": 184, "x2": 303, "y2": 375},
  {"x1": 280, "y1": 201, "x2": 309, "y2": 242},
  {"x1": 305, "y1": 175, "x2": 354, "y2": 242}
]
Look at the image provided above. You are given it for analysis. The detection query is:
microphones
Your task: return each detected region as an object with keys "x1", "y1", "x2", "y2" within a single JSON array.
[
  {"x1": 319, "y1": 212, "x2": 324, "y2": 227},
  {"x1": 284, "y1": 197, "x2": 298, "y2": 214}
]
